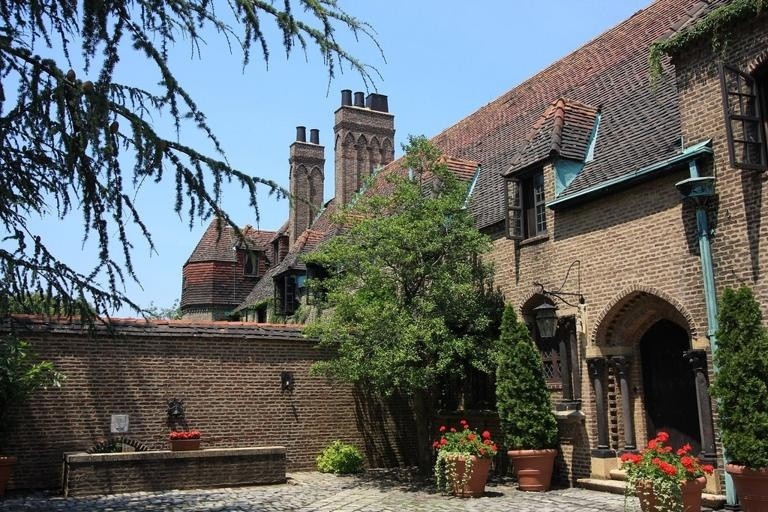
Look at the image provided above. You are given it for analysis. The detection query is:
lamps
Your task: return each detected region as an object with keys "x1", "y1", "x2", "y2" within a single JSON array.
[
  {"x1": 281, "y1": 370, "x2": 295, "y2": 390},
  {"x1": 531, "y1": 282, "x2": 584, "y2": 339}
]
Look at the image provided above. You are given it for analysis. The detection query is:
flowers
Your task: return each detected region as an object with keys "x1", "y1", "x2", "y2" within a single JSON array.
[
  {"x1": 169, "y1": 428, "x2": 201, "y2": 439},
  {"x1": 619, "y1": 430, "x2": 714, "y2": 512},
  {"x1": 431, "y1": 419, "x2": 498, "y2": 496}
]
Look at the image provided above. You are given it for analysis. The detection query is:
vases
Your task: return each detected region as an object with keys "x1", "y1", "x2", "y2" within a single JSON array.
[
  {"x1": 168, "y1": 438, "x2": 200, "y2": 451},
  {"x1": 634, "y1": 476, "x2": 707, "y2": 511},
  {"x1": 443, "y1": 453, "x2": 491, "y2": 498}
]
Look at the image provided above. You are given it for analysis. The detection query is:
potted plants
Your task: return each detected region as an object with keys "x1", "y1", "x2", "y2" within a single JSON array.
[
  {"x1": 494, "y1": 300, "x2": 559, "y2": 492},
  {"x1": 706, "y1": 281, "x2": 767, "y2": 512}
]
